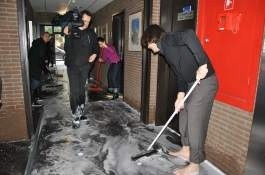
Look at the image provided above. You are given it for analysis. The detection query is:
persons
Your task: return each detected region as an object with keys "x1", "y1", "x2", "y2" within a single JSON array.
[
  {"x1": 97, "y1": 37, "x2": 120, "y2": 101},
  {"x1": 141, "y1": 24, "x2": 218, "y2": 175},
  {"x1": 28, "y1": 31, "x2": 52, "y2": 106},
  {"x1": 63, "y1": 9, "x2": 100, "y2": 124}
]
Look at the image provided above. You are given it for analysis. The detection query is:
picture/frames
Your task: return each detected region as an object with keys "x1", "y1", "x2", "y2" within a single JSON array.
[{"x1": 127, "y1": 11, "x2": 143, "y2": 52}]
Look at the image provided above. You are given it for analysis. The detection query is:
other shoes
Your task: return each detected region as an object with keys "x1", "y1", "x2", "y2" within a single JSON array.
[
  {"x1": 72, "y1": 115, "x2": 80, "y2": 127},
  {"x1": 80, "y1": 110, "x2": 87, "y2": 120}
]
